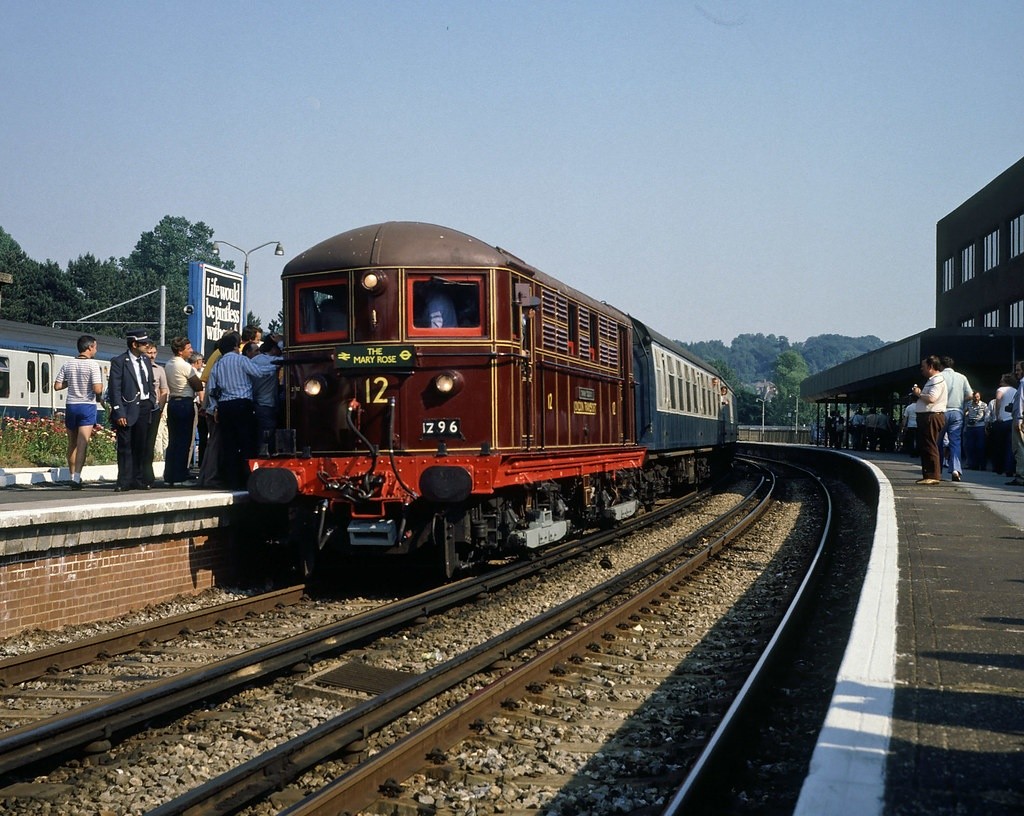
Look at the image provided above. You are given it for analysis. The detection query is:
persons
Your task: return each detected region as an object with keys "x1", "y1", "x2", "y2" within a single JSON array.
[
  {"x1": 936, "y1": 356, "x2": 974, "y2": 481},
  {"x1": 314, "y1": 299, "x2": 342, "y2": 331},
  {"x1": 1004, "y1": 361, "x2": 1024, "y2": 485},
  {"x1": 826, "y1": 390, "x2": 997, "y2": 471},
  {"x1": 99, "y1": 382, "x2": 118, "y2": 432},
  {"x1": 418, "y1": 279, "x2": 458, "y2": 328},
  {"x1": 187, "y1": 326, "x2": 286, "y2": 487},
  {"x1": 911, "y1": 356, "x2": 948, "y2": 484},
  {"x1": 142, "y1": 341, "x2": 167, "y2": 480},
  {"x1": 996, "y1": 374, "x2": 1018, "y2": 477},
  {"x1": 164, "y1": 336, "x2": 202, "y2": 487},
  {"x1": 108, "y1": 328, "x2": 156, "y2": 492},
  {"x1": 55, "y1": 335, "x2": 102, "y2": 490},
  {"x1": 513, "y1": 296, "x2": 541, "y2": 342}
]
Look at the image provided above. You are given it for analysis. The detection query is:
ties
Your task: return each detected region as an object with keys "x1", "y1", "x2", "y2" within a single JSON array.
[{"x1": 136, "y1": 358, "x2": 150, "y2": 396}]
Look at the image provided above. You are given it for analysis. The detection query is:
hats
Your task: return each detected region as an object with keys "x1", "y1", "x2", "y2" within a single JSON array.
[
  {"x1": 523, "y1": 296, "x2": 542, "y2": 311},
  {"x1": 126, "y1": 328, "x2": 153, "y2": 343}
]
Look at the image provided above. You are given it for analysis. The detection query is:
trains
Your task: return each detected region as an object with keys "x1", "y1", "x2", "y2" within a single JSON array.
[
  {"x1": 0, "y1": 319, "x2": 174, "y2": 422},
  {"x1": 247, "y1": 218, "x2": 739, "y2": 584}
]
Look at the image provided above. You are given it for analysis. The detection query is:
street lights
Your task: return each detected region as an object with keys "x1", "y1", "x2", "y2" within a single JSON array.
[
  {"x1": 989, "y1": 327, "x2": 1015, "y2": 373},
  {"x1": 789, "y1": 393, "x2": 801, "y2": 444},
  {"x1": 756, "y1": 397, "x2": 772, "y2": 441},
  {"x1": 211, "y1": 240, "x2": 284, "y2": 328}
]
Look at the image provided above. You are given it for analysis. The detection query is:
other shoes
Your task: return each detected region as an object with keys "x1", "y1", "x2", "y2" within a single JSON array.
[
  {"x1": 164, "y1": 482, "x2": 172, "y2": 487},
  {"x1": 69, "y1": 480, "x2": 82, "y2": 491},
  {"x1": 137, "y1": 483, "x2": 150, "y2": 490},
  {"x1": 942, "y1": 457, "x2": 1017, "y2": 478},
  {"x1": 173, "y1": 480, "x2": 198, "y2": 488},
  {"x1": 952, "y1": 470, "x2": 961, "y2": 482},
  {"x1": 916, "y1": 478, "x2": 940, "y2": 485},
  {"x1": 114, "y1": 484, "x2": 128, "y2": 492},
  {"x1": 1005, "y1": 479, "x2": 1024, "y2": 486}
]
